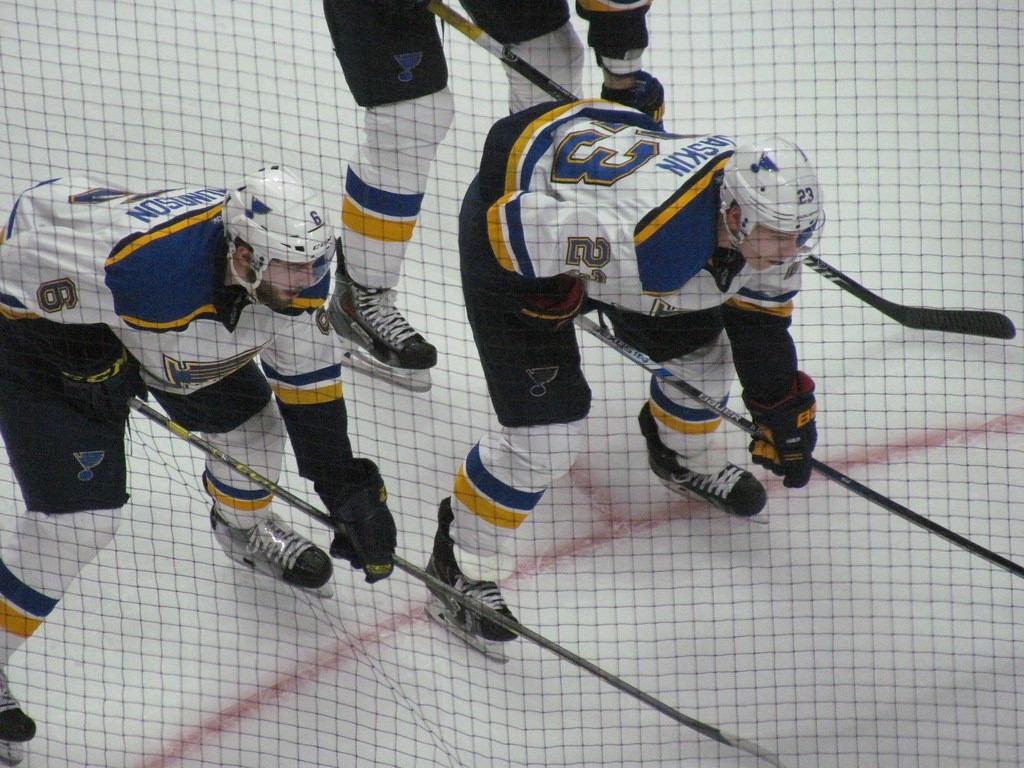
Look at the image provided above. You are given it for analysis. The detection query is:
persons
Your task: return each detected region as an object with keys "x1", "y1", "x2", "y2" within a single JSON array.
[
  {"x1": 425, "y1": 101, "x2": 825, "y2": 642},
  {"x1": 319, "y1": 0, "x2": 666, "y2": 372},
  {"x1": 0, "y1": 164, "x2": 398, "y2": 743}
]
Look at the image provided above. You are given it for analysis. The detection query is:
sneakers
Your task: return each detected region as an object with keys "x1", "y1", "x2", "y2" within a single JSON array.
[
  {"x1": 328, "y1": 236, "x2": 437, "y2": 392},
  {"x1": 0, "y1": 670, "x2": 35, "y2": 766},
  {"x1": 425, "y1": 495, "x2": 520, "y2": 659},
  {"x1": 637, "y1": 400, "x2": 770, "y2": 524},
  {"x1": 209, "y1": 505, "x2": 334, "y2": 600}
]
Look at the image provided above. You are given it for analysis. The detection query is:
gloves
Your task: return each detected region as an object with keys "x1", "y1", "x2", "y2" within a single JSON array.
[
  {"x1": 314, "y1": 457, "x2": 397, "y2": 585},
  {"x1": 518, "y1": 278, "x2": 593, "y2": 338},
  {"x1": 742, "y1": 371, "x2": 818, "y2": 487},
  {"x1": 601, "y1": 70, "x2": 665, "y2": 129},
  {"x1": 59, "y1": 326, "x2": 149, "y2": 420}
]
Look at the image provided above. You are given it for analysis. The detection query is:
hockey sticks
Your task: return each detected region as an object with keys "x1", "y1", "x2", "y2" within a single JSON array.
[
  {"x1": 127, "y1": 396, "x2": 784, "y2": 767},
  {"x1": 573, "y1": 314, "x2": 1024, "y2": 576},
  {"x1": 430, "y1": 0, "x2": 1016, "y2": 340}
]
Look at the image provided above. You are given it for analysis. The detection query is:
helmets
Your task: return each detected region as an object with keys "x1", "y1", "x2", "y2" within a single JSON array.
[
  {"x1": 720, "y1": 137, "x2": 827, "y2": 273},
  {"x1": 225, "y1": 165, "x2": 336, "y2": 315}
]
